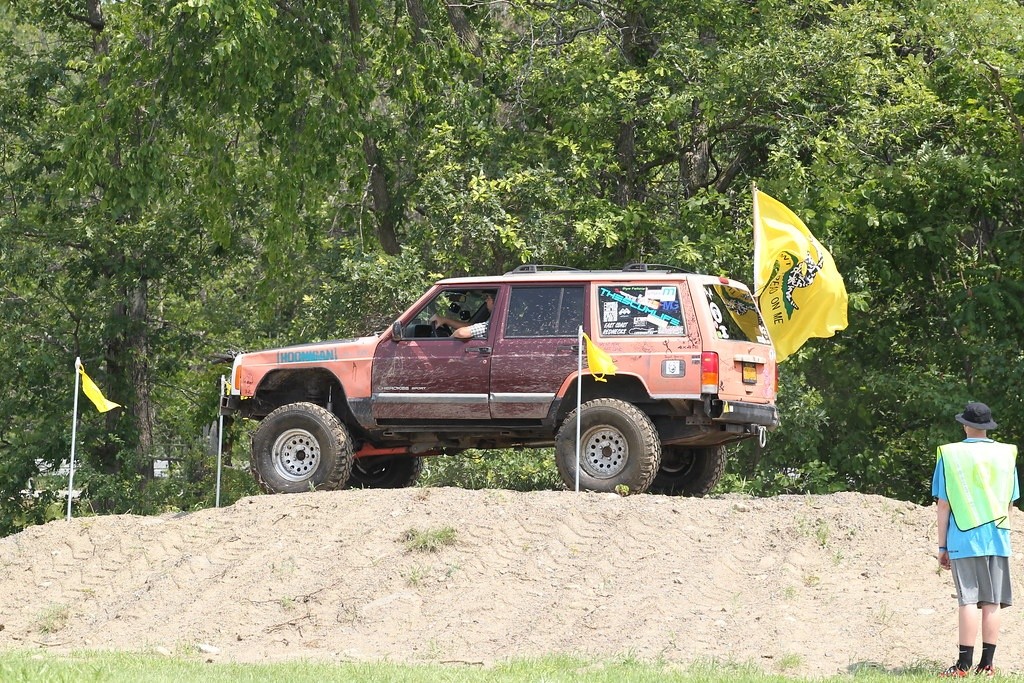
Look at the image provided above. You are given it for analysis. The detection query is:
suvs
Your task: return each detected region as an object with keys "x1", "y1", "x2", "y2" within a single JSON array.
[{"x1": 230, "y1": 262, "x2": 781, "y2": 496}]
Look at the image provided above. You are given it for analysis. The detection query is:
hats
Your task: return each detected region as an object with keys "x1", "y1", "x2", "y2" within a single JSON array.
[
  {"x1": 955, "y1": 402, "x2": 998, "y2": 430},
  {"x1": 482, "y1": 289, "x2": 496, "y2": 295}
]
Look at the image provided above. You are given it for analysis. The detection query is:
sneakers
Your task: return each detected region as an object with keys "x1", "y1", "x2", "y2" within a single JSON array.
[
  {"x1": 971, "y1": 665, "x2": 994, "y2": 679},
  {"x1": 937, "y1": 664, "x2": 973, "y2": 679}
]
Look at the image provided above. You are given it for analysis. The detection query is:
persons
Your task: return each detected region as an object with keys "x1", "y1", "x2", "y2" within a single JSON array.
[
  {"x1": 930, "y1": 401, "x2": 1019, "y2": 678},
  {"x1": 430, "y1": 291, "x2": 498, "y2": 340}
]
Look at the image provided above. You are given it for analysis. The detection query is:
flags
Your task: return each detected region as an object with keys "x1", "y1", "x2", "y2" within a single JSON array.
[{"x1": 758, "y1": 189, "x2": 849, "y2": 364}]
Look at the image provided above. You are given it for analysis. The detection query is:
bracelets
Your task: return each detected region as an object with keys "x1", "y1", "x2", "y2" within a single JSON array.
[{"x1": 939, "y1": 547, "x2": 946, "y2": 553}]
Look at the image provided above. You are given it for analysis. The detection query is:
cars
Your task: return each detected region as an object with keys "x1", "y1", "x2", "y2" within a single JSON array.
[{"x1": 152, "y1": 459, "x2": 181, "y2": 480}]
